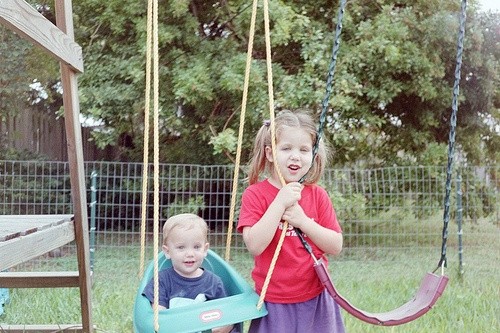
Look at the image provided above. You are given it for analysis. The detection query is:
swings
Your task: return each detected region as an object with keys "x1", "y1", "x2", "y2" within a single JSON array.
[
  {"x1": 292, "y1": 0, "x2": 468, "y2": 326},
  {"x1": 133, "y1": 0, "x2": 292, "y2": 333}
]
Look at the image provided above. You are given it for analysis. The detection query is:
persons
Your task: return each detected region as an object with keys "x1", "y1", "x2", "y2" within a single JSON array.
[
  {"x1": 141, "y1": 214, "x2": 236, "y2": 333},
  {"x1": 236, "y1": 109, "x2": 349, "y2": 333}
]
